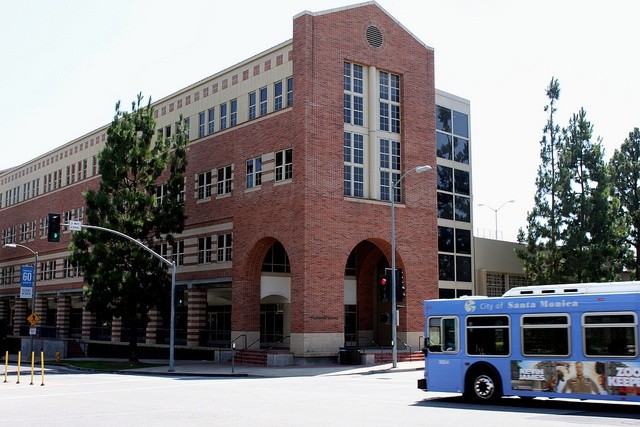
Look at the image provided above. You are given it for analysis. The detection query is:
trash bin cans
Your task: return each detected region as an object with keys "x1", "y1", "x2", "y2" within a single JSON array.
[{"x1": 379, "y1": 312, "x2": 393, "y2": 349}]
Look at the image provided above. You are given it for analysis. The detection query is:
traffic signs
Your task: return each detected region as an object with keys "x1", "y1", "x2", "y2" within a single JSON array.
[{"x1": 20, "y1": 266, "x2": 33, "y2": 285}]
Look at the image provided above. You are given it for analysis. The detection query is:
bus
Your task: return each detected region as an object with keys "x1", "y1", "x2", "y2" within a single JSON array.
[
  {"x1": 417, "y1": 291, "x2": 639, "y2": 404},
  {"x1": 501, "y1": 280, "x2": 639, "y2": 295}
]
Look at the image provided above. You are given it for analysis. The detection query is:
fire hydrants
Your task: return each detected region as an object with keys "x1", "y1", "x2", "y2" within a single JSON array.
[{"x1": 55, "y1": 351, "x2": 61, "y2": 361}]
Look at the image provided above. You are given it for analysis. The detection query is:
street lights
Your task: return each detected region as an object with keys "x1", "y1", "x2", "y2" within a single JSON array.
[
  {"x1": 391, "y1": 164, "x2": 431, "y2": 366},
  {"x1": 2, "y1": 243, "x2": 37, "y2": 360},
  {"x1": 477, "y1": 200, "x2": 513, "y2": 238}
]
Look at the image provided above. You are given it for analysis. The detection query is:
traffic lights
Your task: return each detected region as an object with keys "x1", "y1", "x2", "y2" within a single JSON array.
[
  {"x1": 395, "y1": 267, "x2": 405, "y2": 301},
  {"x1": 47, "y1": 212, "x2": 59, "y2": 242},
  {"x1": 378, "y1": 274, "x2": 391, "y2": 301}
]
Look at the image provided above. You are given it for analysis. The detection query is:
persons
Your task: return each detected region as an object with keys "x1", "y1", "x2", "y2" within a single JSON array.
[{"x1": 562, "y1": 362, "x2": 600, "y2": 394}]
